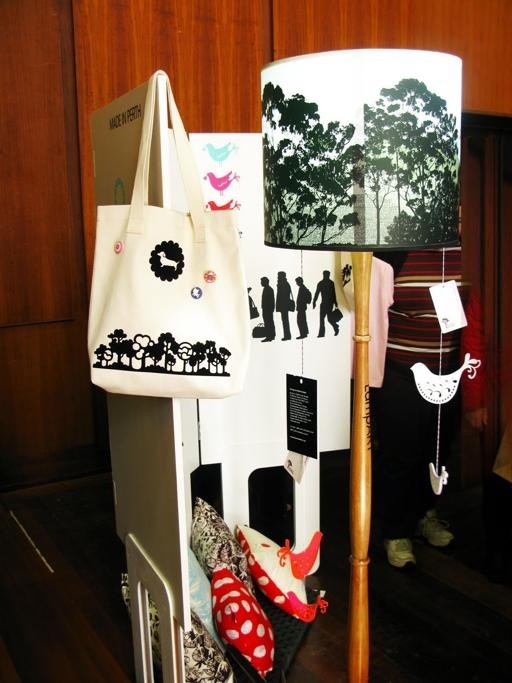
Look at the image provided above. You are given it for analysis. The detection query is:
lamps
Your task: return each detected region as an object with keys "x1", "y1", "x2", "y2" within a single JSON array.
[{"x1": 258, "y1": 43, "x2": 464, "y2": 683}]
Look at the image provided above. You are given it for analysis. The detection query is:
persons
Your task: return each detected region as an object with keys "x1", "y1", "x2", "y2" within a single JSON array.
[
  {"x1": 294, "y1": 276, "x2": 309, "y2": 339},
  {"x1": 312, "y1": 270, "x2": 339, "y2": 338},
  {"x1": 275, "y1": 271, "x2": 291, "y2": 341},
  {"x1": 458, "y1": 287, "x2": 489, "y2": 433},
  {"x1": 373, "y1": 243, "x2": 471, "y2": 573},
  {"x1": 260, "y1": 277, "x2": 276, "y2": 342}
]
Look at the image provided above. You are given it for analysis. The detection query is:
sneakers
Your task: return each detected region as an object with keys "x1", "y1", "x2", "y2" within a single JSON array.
[
  {"x1": 416, "y1": 515, "x2": 455, "y2": 547},
  {"x1": 383, "y1": 536, "x2": 417, "y2": 569}
]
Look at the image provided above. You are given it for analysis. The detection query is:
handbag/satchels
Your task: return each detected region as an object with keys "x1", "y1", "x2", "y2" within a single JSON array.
[{"x1": 84, "y1": 68, "x2": 253, "y2": 402}]
